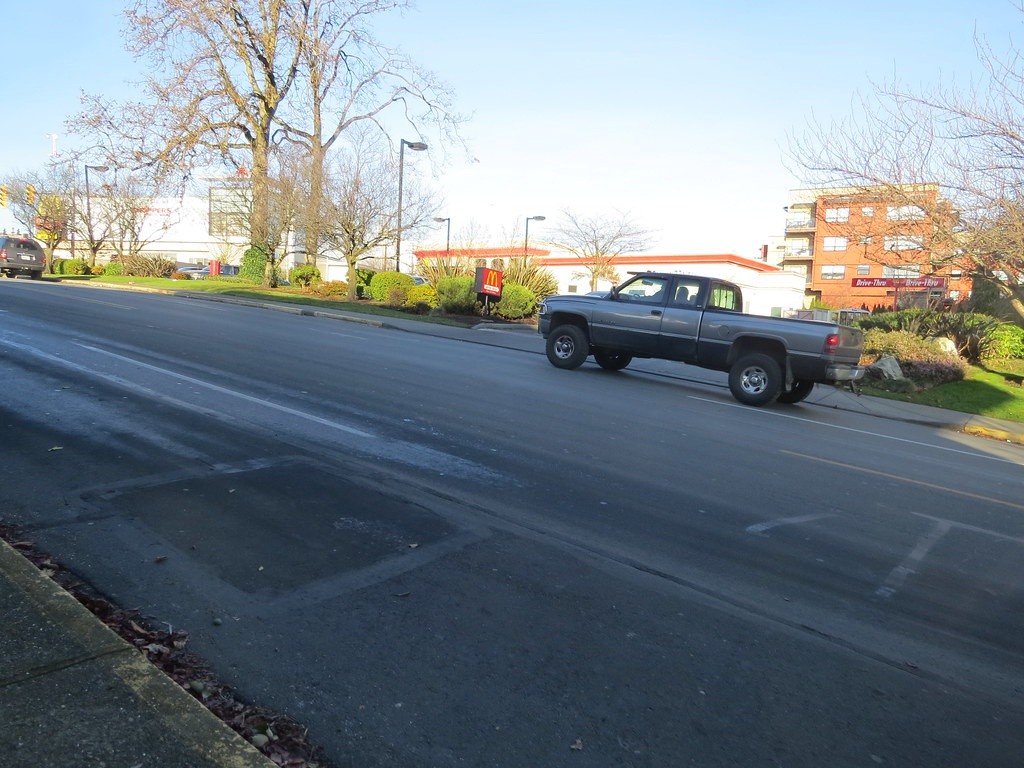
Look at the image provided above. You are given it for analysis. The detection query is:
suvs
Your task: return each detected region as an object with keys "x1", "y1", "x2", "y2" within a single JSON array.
[{"x1": 0, "y1": 235, "x2": 46, "y2": 280}]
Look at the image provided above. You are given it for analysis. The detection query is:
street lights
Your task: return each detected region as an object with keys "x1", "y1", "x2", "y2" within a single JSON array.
[
  {"x1": 396, "y1": 137, "x2": 428, "y2": 272},
  {"x1": 524, "y1": 215, "x2": 546, "y2": 268},
  {"x1": 433, "y1": 217, "x2": 450, "y2": 252},
  {"x1": 83, "y1": 164, "x2": 109, "y2": 220}
]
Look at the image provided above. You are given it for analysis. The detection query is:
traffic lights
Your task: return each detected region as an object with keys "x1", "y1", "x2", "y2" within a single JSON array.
[
  {"x1": 0, "y1": 185, "x2": 7, "y2": 207},
  {"x1": 26, "y1": 185, "x2": 35, "y2": 205}
]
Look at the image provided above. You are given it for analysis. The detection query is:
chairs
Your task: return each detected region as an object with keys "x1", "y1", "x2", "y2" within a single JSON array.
[{"x1": 676, "y1": 287, "x2": 688, "y2": 301}]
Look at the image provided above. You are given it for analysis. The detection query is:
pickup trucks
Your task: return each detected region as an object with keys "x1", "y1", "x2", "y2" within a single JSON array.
[{"x1": 538, "y1": 270, "x2": 866, "y2": 408}]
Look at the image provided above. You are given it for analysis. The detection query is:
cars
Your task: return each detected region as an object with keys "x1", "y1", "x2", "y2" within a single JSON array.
[
  {"x1": 412, "y1": 276, "x2": 431, "y2": 286},
  {"x1": 177, "y1": 264, "x2": 240, "y2": 279},
  {"x1": 586, "y1": 291, "x2": 633, "y2": 298}
]
